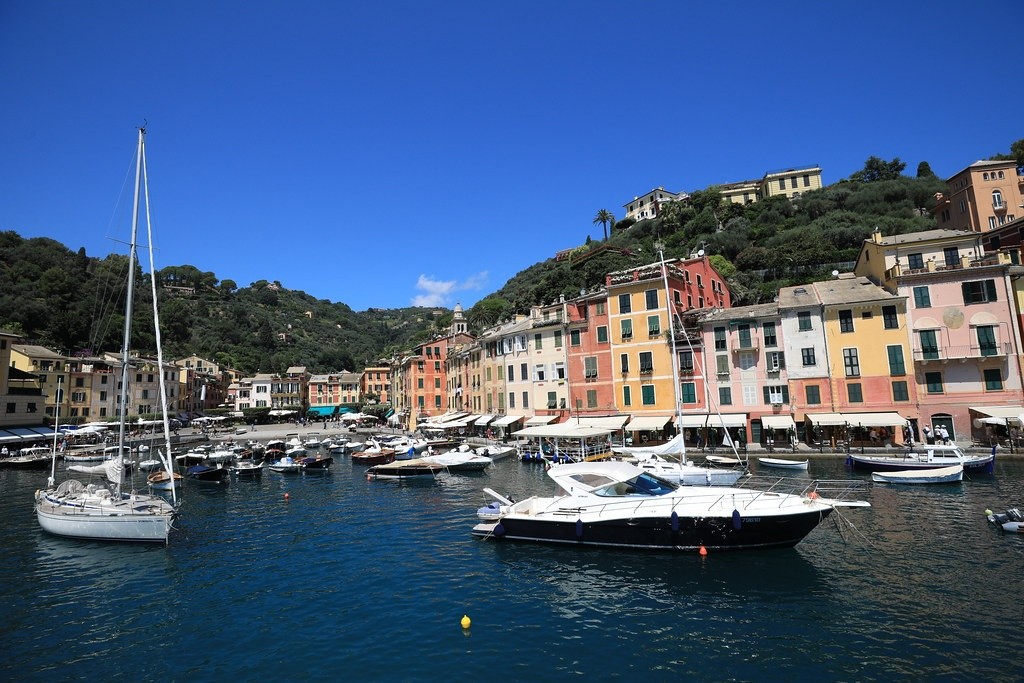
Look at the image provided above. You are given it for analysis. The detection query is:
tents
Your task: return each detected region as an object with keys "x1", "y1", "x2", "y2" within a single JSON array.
[
  {"x1": 339, "y1": 412, "x2": 378, "y2": 429},
  {"x1": 193, "y1": 417, "x2": 229, "y2": 428}
]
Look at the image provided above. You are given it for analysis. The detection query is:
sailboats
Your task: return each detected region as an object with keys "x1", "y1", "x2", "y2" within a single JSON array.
[
  {"x1": 613, "y1": 251, "x2": 749, "y2": 485},
  {"x1": 35, "y1": 119, "x2": 182, "y2": 544}
]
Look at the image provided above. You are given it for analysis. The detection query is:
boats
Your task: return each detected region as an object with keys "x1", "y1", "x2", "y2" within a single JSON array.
[
  {"x1": 64, "y1": 453, "x2": 114, "y2": 462},
  {"x1": 147, "y1": 470, "x2": 185, "y2": 492},
  {"x1": 236, "y1": 429, "x2": 247, "y2": 435},
  {"x1": 51, "y1": 424, "x2": 109, "y2": 435},
  {"x1": 848, "y1": 448, "x2": 993, "y2": 486},
  {"x1": 707, "y1": 455, "x2": 749, "y2": 465},
  {"x1": 474, "y1": 446, "x2": 514, "y2": 461},
  {"x1": 0, "y1": 447, "x2": 66, "y2": 470},
  {"x1": 185, "y1": 466, "x2": 228, "y2": 482},
  {"x1": 473, "y1": 464, "x2": 873, "y2": 548},
  {"x1": 270, "y1": 456, "x2": 334, "y2": 472},
  {"x1": 229, "y1": 462, "x2": 266, "y2": 476},
  {"x1": 139, "y1": 460, "x2": 161, "y2": 470},
  {"x1": 364, "y1": 453, "x2": 493, "y2": 475},
  {"x1": 174, "y1": 438, "x2": 304, "y2": 466},
  {"x1": 320, "y1": 433, "x2": 430, "y2": 463},
  {"x1": 303, "y1": 437, "x2": 322, "y2": 449},
  {"x1": 758, "y1": 458, "x2": 810, "y2": 471}
]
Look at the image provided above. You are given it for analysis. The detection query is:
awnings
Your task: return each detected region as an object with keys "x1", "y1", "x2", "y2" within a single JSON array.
[
  {"x1": 228, "y1": 411, "x2": 247, "y2": 417},
  {"x1": 0, "y1": 420, "x2": 170, "y2": 446},
  {"x1": 338, "y1": 407, "x2": 358, "y2": 414},
  {"x1": 267, "y1": 410, "x2": 298, "y2": 416},
  {"x1": 416, "y1": 411, "x2": 910, "y2": 431},
  {"x1": 305, "y1": 406, "x2": 336, "y2": 417},
  {"x1": 968, "y1": 405, "x2": 1024, "y2": 417},
  {"x1": 384, "y1": 408, "x2": 394, "y2": 418}
]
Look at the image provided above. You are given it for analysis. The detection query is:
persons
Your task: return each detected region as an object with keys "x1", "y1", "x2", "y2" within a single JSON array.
[
  {"x1": 870, "y1": 427, "x2": 888, "y2": 447},
  {"x1": 0, "y1": 428, "x2": 162, "y2": 463},
  {"x1": 412, "y1": 427, "x2": 498, "y2": 457},
  {"x1": 685, "y1": 427, "x2": 745, "y2": 450},
  {"x1": 277, "y1": 416, "x2": 374, "y2": 430},
  {"x1": 986, "y1": 424, "x2": 993, "y2": 447},
  {"x1": 1011, "y1": 426, "x2": 1019, "y2": 437},
  {"x1": 923, "y1": 424, "x2": 950, "y2": 444},
  {"x1": 198, "y1": 427, "x2": 219, "y2": 439},
  {"x1": 526, "y1": 436, "x2": 606, "y2": 450}
]
